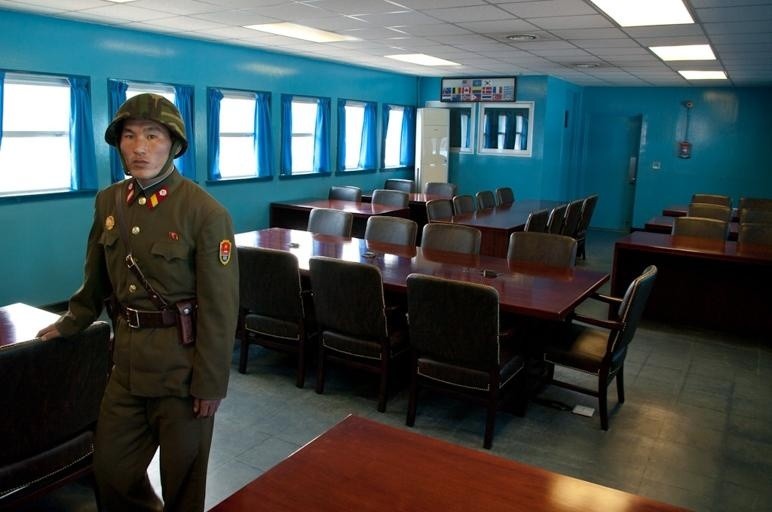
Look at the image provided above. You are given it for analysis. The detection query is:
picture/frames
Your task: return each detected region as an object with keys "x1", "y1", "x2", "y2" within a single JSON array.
[{"x1": 440, "y1": 76, "x2": 516, "y2": 102}]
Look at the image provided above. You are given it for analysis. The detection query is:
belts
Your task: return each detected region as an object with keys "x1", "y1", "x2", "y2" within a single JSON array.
[{"x1": 116, "y1": 304, "x2": 174, "y2": 330}]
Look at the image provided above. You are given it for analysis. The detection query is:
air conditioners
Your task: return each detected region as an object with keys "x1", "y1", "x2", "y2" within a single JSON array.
[{"x1": 415, "y1": 107, "x2": 451, "y2": 192}]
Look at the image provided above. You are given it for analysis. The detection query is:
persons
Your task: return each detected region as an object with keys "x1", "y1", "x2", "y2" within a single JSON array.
[{"x1": 37, "y1": 93, "x2": 240, "y2": 512}]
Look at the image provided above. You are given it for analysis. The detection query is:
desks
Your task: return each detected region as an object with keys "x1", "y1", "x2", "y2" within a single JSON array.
[
  {"x1": 205, "y1": 413, "x2": 700, "y2": 512},
  {"x1": 224, "y1": 226, "x2": 611, "y2": 413},
  {"x1": 0, "y1": 302, "x2": 115, "y2": 346}
]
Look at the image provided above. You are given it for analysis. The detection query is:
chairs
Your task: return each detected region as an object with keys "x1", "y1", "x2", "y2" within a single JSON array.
[
  {"x1": 608, "y1": 192, "x2": 772, "y2": 353},
  {"x1": 506, "y1": 231, "x2": 579, "y2": 266},
  {"x1": 404, "y1": 273, "x2": 531, "y2": 450},
  {"x1": 531, "y1": 263, "x2": 658, "y2": 431},
  {"x1": 364, "y1": 216, "x2": 419, "y2": 246},
  {"x1": 425, "y1": 187, "x2": 598, "y2": 260},
  {"x1": 306, "y1": 208, "x2": 354, "y2": 238},
  {"x1": 421, "y1": 223, "x2": 482, "y2": 254},
  {"x1": 237, "y1": 245, "x2": 318, "y2": 389},
  {"x1": 269, "y1": 179, "x2": 457, "y2": 247},
  {"x1": 305, "y1": 255, "x2": 424, "y2": 413},
  {"x1": 0, "y1": 320, "x2": 113, "y2": 509}
]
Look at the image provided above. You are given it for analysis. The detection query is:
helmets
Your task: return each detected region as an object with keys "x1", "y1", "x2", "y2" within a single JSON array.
[{"x1": 105, "y1": 93, "x2": 188, "y2": 159}]
